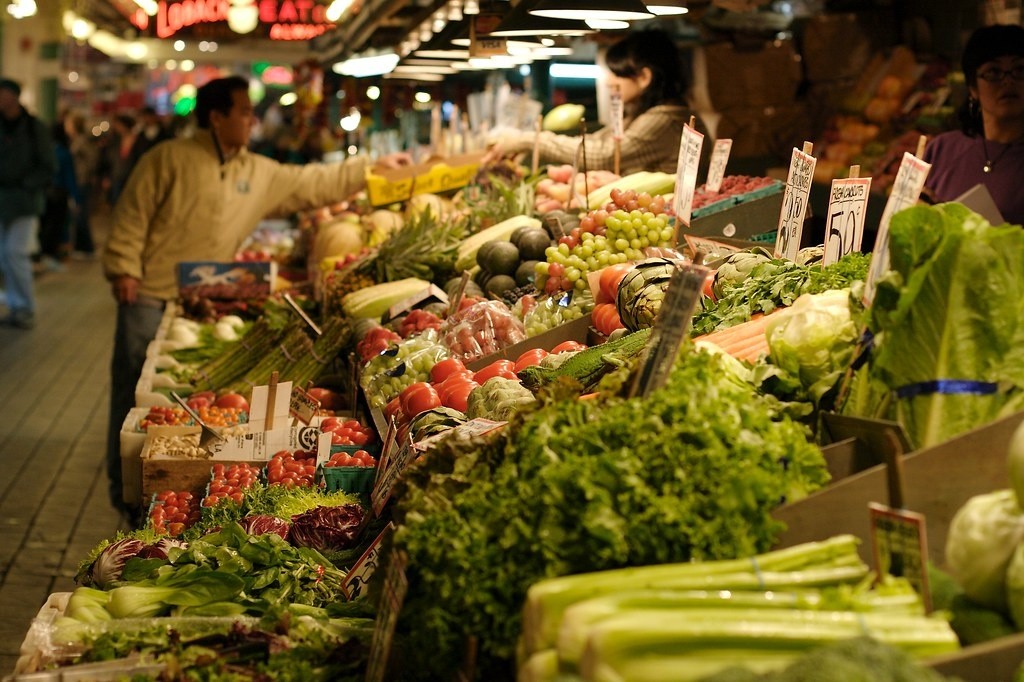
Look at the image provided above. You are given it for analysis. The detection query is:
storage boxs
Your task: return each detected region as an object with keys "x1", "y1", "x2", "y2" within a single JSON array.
[{"x1": 17, "y1": 36, "x2": 1024, "y2": 682}]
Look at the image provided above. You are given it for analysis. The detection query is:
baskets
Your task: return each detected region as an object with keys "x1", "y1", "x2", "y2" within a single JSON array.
[
  {"x1": 316, "y1": 444, "x2": 377, "y2": 501},
  {"x1": 690, "y1": 179, "x2": 785, "y2": 241}
]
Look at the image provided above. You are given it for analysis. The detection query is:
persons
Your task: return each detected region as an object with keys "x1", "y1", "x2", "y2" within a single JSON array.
[
  {"x1": 483, "y1": 29, "x2": 710, "y2": 185},
  {"x1": 31, "y1": 106, "x2": 197, "y2": 274},
  {"x1": 916, "y1": 23, "x2": 1024, "y2": 232},
  {"x1": 0, "y1": 79, "x2": 52, "y2": 329},
  {"x1": 249, "y1": 101, "x2": 358, "y2": 164},
  {"x1": 103, "y1": 76, "x2": 413, "y2": 500}
]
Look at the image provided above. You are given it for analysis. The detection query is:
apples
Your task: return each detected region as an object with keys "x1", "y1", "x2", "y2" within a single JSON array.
[{"x1": 235, "y1": 249, "x2": 280, "y2": 261}]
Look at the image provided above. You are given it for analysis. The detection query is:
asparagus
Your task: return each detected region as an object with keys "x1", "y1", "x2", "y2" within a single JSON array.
[{"x1": 188, "y1": 305, "x2": 351, "y2": 391}]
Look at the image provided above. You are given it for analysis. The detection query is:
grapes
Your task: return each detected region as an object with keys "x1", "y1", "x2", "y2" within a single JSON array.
[{"x1": 359, "y1": 187, "x2": 675, "y2": 404}]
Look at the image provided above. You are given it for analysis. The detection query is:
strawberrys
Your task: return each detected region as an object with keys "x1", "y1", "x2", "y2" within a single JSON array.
[{"x1": 661, "y1": 174, "x2": 774, "y2": 215}]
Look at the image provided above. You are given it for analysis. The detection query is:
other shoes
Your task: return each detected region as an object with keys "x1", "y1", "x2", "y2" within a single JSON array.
[
  {"x1": 0, "y1": 307, "x2": 35, "y2": 330},
  {"x1": 110, "y1": 486, "x2": 140, "y2": 526}
]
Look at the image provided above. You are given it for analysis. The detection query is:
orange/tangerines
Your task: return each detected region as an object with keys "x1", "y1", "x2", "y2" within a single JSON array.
[{"x1": 814, "y1": 76, "x2": 903, "y2": 185}]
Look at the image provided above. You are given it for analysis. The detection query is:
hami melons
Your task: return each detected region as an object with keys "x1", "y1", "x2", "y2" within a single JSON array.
[{"x1": 306, "y1": 193, "x2": 453, "y2": 266}]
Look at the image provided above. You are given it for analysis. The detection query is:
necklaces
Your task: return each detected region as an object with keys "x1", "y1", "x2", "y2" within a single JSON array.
[{"x1": 983, "y1": 135, "x2": 1017, "y2": 172}]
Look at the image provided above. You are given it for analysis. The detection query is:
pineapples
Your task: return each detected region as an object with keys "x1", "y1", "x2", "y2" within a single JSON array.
[{"x1": 328, "y1": 210, "x2": 464, "y2": 307}]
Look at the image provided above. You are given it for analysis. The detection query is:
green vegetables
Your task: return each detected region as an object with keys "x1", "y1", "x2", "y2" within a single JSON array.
[
  {"x1": 698, "y1": 198, "x2": 1024, "y2": 454},
  {"x1": 29, "y1": 482, "x2": 446, "y2": 682},
  {"x1": 392, "y1": 335, "x2": 963, "y2": 682}
]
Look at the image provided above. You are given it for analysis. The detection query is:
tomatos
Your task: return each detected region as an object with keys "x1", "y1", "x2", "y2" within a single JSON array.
[
  {"x1": 135, "y1": 386, "x2": 379, "y2": 534},
  {"x1": 383, "y1": 259, "x2": 720, "y2": 444}
]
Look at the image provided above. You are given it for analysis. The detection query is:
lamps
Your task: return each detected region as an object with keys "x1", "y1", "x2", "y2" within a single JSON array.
[{"x1": 395, "y1": 0, "x2": 689, "y2": 74}]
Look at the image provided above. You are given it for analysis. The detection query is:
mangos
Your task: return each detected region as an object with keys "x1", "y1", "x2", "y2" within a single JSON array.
[{"x1": 532, "y1": 165, "x2": 623, "y2": 212}]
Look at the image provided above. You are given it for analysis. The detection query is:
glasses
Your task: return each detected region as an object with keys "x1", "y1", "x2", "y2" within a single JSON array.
[{"x1": 974, "y1": 66, "x2": 1024, "y2": 83}]
